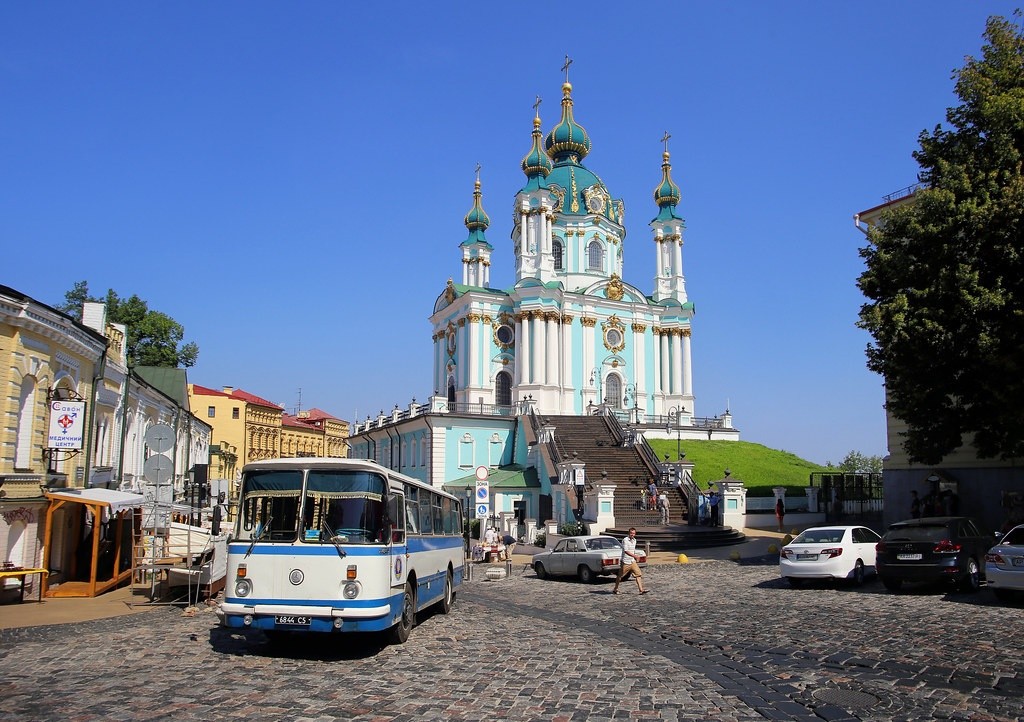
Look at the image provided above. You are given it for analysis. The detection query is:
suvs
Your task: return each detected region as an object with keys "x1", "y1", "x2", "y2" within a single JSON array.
[{"x1": 876, "y1": 517, "x2": 1003, "y2": 592}]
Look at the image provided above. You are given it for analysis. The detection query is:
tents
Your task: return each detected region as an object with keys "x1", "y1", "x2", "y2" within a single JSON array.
[{"x1": 41, "y1": 485, "x2": 148, "y2": 602}]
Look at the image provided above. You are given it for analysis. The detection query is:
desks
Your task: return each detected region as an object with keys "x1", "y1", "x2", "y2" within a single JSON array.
[{"x1": 0, "y1": 568, "x2": 49, "y2": 602}]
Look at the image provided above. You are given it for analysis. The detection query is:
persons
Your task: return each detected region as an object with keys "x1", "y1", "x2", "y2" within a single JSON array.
[
  {"x1": 831, "y1": 496, "x2": 845, "y2": 527},
  {"x1": 910, "y1": 490, "x2": 921, "y2": 519},
  {"x1": 612, "y1": 527, "x2": 652, "y2": 596},
  {"x1": 646, "y1": 478, "x2": 660, "y2": 511},
  {"x1": 920, "y1": 487, "x2": 964, "y2": 519},
  {"x1": 701, "y1": 490, "x2": 723, "y2": 528},
  {"x1": 639, "y1": 486, "x2": 649, "y2": 511},
  {"x1": 377, "y1": 511, "x2": 399, "y2": 544},
  {"x1": 498, "y1": 534, "x2": 516, "y2": 561},
  {"x1": 774, "y1": 497, "x2": 786, "y2": 533}
]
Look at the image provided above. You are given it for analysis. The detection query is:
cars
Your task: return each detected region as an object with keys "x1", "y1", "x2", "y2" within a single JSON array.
[
  {"x1": 781, "y1": 526, "x2": 882, "y2": 588},
  {"x1": 985, "y1": 525, "x2": 1024, "y2": 591},
  {"x1": 530, "y1": 536, "x2": 647, "y2": 584}
]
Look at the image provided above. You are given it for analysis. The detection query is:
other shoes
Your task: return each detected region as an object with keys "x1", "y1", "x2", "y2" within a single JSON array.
[
  {"x1": 612, "y1": 590, "x2": 622, "y2": 594},
  {"x1": 639, "y1": 589, "x2": 651, "y2": 594}
]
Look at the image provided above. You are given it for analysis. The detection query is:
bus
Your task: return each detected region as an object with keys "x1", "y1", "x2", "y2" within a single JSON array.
[{"x1": 212, "y1": 458, "x2": 466, "y2": 644}]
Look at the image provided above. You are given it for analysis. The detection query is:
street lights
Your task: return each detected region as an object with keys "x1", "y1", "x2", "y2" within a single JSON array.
[
  {"x1": 623, "y1": 383, "x2": 638, "y2": 426},
  {"x1": 666, "y1": 405, "x2": 681, "y2": 461},
  {"x1": 188, "y1": 465, "x2": 196, "y2": 526}
]
